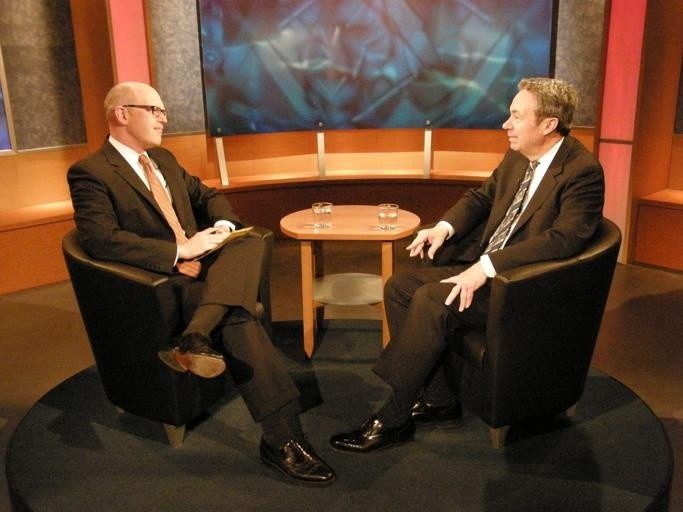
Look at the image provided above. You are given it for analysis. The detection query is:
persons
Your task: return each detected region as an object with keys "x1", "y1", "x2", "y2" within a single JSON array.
[
  {"x1": 67, "y1": 82, "x2": 337, "y2": 487},
  {"x1": 329, "y1": 77, "x2": 606, "y2": 454}
]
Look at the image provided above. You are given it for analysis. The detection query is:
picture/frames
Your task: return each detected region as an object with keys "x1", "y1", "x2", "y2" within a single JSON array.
[{"x1": 192, "y1": 0, "x2": 560, "y2": 137}]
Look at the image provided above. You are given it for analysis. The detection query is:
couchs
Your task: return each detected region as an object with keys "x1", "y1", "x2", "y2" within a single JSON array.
[
  {"x1": 413, "y1": 217, "x2": 624, "y2": 446},
  {"x1": 63, "y1": 227, "x2": 274, "y2": 449}
]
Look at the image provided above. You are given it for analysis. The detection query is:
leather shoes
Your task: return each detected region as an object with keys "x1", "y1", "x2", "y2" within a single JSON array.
[
  {"x1": 327, "y1": 415, "x2": 416, "y2": 454},
  {"x1": 409, "y1": 397, "x2": 463, "y2": 431},
  {"x1": 259, "y1": 434, "x2": 337, "y2": 487},
  {"x1": 158, "y1": 332, "x2": 226, "y2": 378}
]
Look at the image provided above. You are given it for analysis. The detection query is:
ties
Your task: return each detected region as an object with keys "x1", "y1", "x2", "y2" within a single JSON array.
[
  {"x1": 483, "y1": 161, "x2": 538, "y2": 252},
  {"x1": 138, "y1": 155, "x2": 189, "y2": 246}
]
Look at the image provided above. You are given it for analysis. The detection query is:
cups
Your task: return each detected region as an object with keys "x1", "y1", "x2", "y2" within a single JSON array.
[
  {"x1": 377, "y1": 203, "x2": 398, "y2": 235},
  {"x1": 312, "y1": 202, "x2": 332, "y2": 233}
]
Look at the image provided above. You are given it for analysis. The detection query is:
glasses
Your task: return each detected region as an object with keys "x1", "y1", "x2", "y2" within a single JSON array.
[{"x1": 117, "y1": 105, "x2": 167, "y2": 118}]
